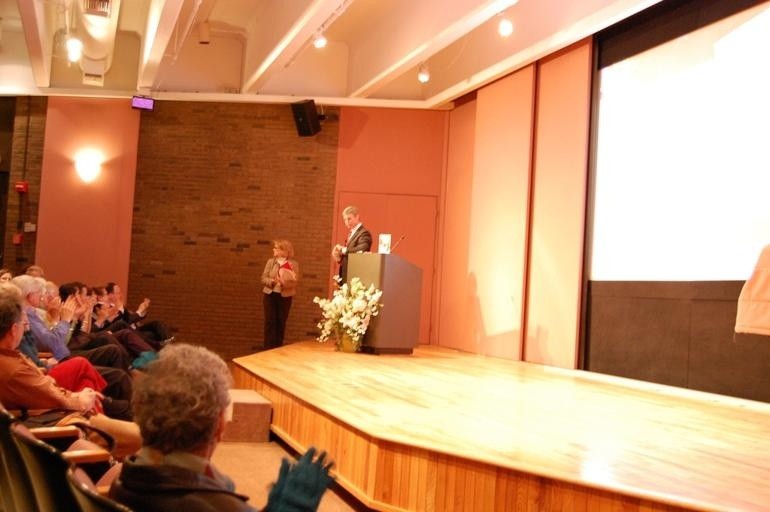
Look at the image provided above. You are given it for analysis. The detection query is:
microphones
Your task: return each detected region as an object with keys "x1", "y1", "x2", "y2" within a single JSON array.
[{"x1": 390, "y1": 235, "x2": 405, "y2": 253}]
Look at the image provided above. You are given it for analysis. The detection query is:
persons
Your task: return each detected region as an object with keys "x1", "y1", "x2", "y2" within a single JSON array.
[
  {"x1": 106, "y1": 342, "x2": 337, "y2": 511},
  {"x1": 331, "y1": 206, "x2": 372, "y2": 288},
  {"x1": 260, "y1": 239, "x2": 299, "y2": 351},
  {"x1": 1, "y1": 266, "x2": 178, "y2": 462}
]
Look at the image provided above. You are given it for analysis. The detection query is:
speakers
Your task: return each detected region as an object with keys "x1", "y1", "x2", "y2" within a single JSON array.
[{"x1": 291, "y1": 100, "x2": 322, "y2": 136}]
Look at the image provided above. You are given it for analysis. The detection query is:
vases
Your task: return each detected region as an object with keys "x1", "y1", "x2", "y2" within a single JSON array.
[{"x1": 335, "y1": 327, "x2": 360, "y2": 353}]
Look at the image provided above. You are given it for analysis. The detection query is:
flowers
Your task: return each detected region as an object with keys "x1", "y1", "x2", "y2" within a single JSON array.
[{"x1": 313, "y1": 276, "x2": 383, "y2": 344}]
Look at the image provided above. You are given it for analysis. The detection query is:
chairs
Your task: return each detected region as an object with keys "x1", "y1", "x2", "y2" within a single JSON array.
[
  {"x1": 0, "y1": 406, "x2": 39, "y2": 512},
  {"x1": 65, "y1": 459, "x2": 131, "y2": 512},
  {"x1": 12, "y1": 422, "x2": 111, "y2": 512}
]
